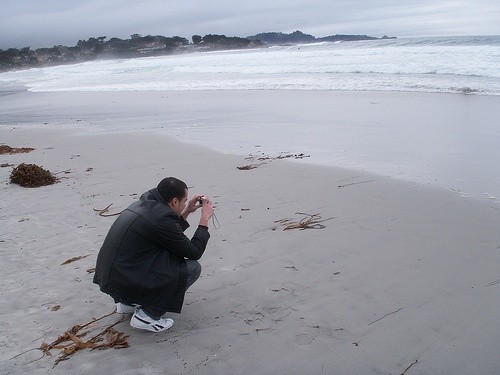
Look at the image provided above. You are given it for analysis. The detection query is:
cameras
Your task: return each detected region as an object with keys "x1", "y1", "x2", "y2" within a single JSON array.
[{"x1": 199, "y1": 196, "x2": 205, "y2": 205}]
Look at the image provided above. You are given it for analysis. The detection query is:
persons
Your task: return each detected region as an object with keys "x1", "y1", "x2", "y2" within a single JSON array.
[{"x1": 93, "y1": 176, "x2": 214, "y2": 332}]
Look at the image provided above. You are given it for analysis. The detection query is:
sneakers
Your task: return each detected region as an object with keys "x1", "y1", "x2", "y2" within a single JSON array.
[
  {"x1": 130, "y1": 308, "x2": 174, "y2": 332},
  {"x1": 116, "y1": 302, "x2": 141, "y2": 314}
]
